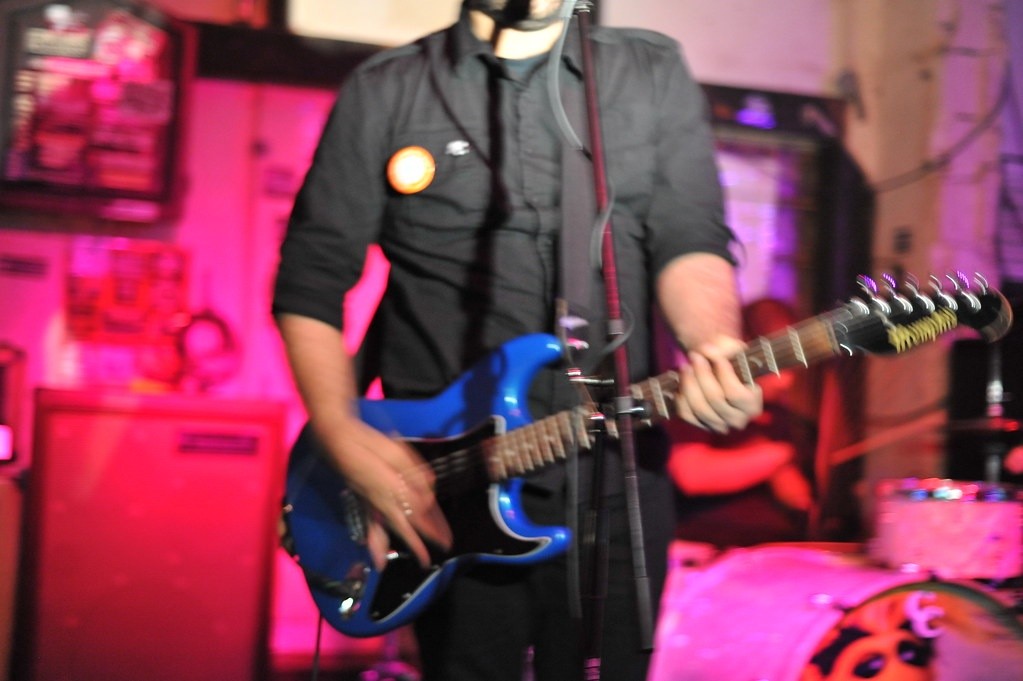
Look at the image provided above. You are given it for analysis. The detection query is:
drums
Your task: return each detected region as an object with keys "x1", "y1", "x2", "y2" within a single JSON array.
[
  {"x1": 648, "y1": 544, "x2": 1023, "y2": 680},
  {"x1": 877, "y1": 472, "x2": 1022, "y2": 580}
]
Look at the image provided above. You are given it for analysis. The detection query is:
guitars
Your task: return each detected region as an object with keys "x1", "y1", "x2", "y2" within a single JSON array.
[{"x1": 281, "y1": 270, "x2": 1015, "y2": 640}]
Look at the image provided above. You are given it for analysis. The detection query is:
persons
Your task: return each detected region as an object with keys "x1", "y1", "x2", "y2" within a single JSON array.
[
  {"x1": 270, "y1": 0, "x2": 762, "y2": 681},
  {"x1": 667, "y1": 300, "x2": 816, "y2": 550}
]
